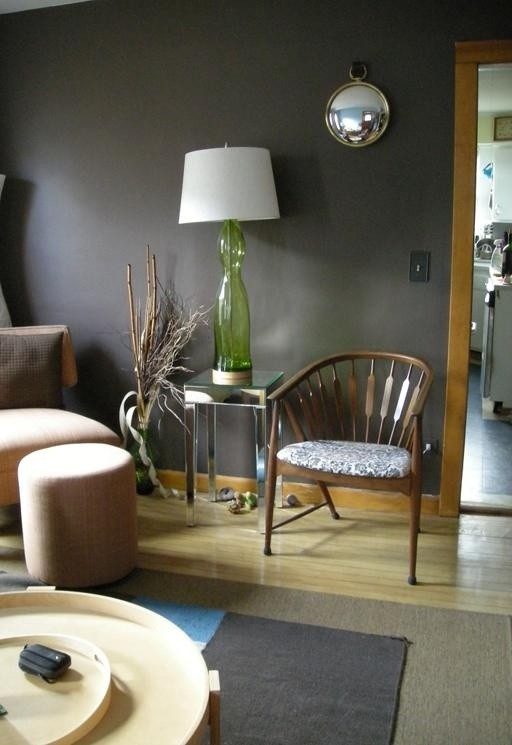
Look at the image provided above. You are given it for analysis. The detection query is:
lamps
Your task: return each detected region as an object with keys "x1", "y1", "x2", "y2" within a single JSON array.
[{"x1": 176, "y1": 142, "x2": 281, "y2": 388}]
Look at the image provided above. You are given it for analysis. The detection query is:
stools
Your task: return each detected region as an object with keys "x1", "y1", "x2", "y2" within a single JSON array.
[{"x1": 18, "y1": 443, "x2": 140, "y2": 589}]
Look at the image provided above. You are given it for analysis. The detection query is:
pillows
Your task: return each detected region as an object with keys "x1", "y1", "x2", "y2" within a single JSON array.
[{"x1": 1, "y1": 331, "x2": 65, "y2": 411}]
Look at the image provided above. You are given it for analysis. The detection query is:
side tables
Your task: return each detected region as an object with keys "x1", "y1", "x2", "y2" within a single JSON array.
[{"x1": 183, "y1": 368, "x2": 284, "y2": 533}]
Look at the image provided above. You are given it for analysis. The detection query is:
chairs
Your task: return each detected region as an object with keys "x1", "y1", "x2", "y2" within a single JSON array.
[
  {"x1": 262, "y1": 349, "x2": 434, "y2": 587},
  {"x1": 0, "y1": 324, "x2": 122, "y2": 507}
]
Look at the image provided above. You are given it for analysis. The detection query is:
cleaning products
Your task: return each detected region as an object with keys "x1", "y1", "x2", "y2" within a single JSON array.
[{"x1": 488, "y1": 239, "x2": 505, "y2": 277}]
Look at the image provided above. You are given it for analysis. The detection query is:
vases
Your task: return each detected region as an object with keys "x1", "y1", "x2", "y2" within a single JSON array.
[{"x1": 131, "y1": 429, "x2": 160, "y2": 496}]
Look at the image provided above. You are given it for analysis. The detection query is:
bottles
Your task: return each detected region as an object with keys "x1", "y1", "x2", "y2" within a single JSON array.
[{"x1": 501, "y1": 232, "x2": 512, "y2": 279}]
